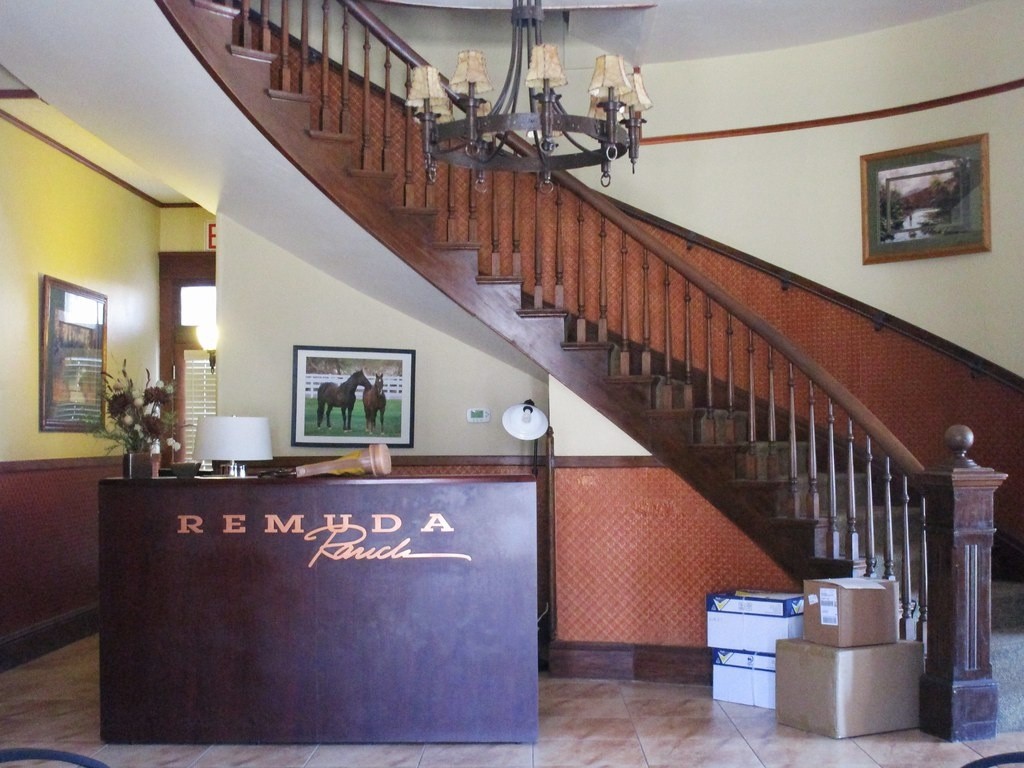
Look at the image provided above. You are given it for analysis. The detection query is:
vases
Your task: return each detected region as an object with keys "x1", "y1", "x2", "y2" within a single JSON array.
[{"x1": 123, "y1": 452, "x2": 161, "y2": 477}]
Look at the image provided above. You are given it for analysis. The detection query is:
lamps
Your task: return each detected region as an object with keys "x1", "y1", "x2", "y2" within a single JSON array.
[
  {"x1": 503, "y1": 397, "x2": 549, "y2": 480},
  {"x1": 196, "y1": 323, "x2": 220, "y2": 374},
  {"x1": 191, "y1": 414, "x2": 273, "y2": 480},
  {"x1": 405, "y1": 0, "x2": 651, "y2": 194}
]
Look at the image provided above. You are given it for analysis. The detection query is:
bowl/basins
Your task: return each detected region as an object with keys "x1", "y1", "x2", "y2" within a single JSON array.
[{"x1": 171, "y1": 461, "x2": 202, "y2": 479}]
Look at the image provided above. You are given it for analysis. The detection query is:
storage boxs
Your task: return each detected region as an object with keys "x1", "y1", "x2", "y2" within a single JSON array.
[{"x1": 706, "y1": 576, "x2": 924, "y2": 739}]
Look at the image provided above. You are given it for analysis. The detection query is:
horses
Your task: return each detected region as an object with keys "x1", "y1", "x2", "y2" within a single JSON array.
[
  {"x1": 316, "y1": 367, "x2": 372, "y2": 433},
  {"x1": 362, "y1": 374, "x2": 386, "y2": 436}
]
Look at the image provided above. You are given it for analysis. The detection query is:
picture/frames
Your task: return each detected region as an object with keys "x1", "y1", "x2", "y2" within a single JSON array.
[
  {"x1": 291, "y1": 343, "x2": 416, "y2": 448},
  {"x1": 39, "y1": 274, "x2": 109, "y2": 433},
  {"x1": 859, "y1": 133, "x2": 993, "y2": 265}
]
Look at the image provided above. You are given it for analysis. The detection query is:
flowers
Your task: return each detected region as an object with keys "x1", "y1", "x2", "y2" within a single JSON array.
[{"x1": 89, "y1": 358, "x2": 196, "y2": 453}]
